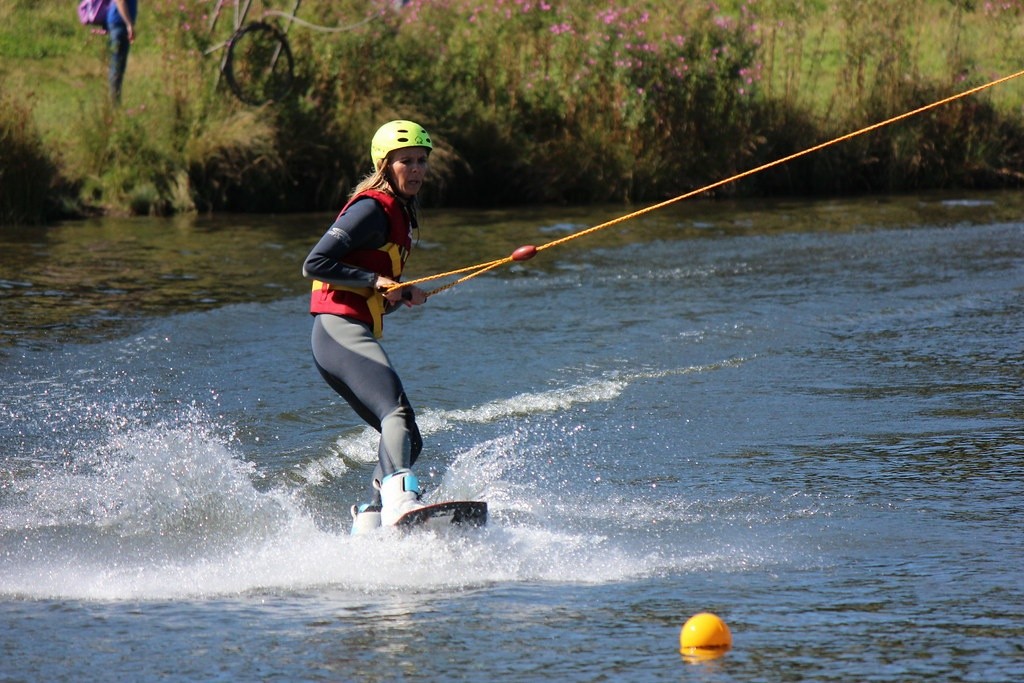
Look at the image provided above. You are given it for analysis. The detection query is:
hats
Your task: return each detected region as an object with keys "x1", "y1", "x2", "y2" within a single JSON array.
[{"x1": 370, "y1": 119, "x2": 435, "y2": 168}]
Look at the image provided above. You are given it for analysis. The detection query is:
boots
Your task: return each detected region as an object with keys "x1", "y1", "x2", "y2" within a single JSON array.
[
  {"x1": 370, "y1": 471, "x2": 430, "y2": 528},
  {"x1": 346, "y1": 501, "x2": 383, "y2": 535}
]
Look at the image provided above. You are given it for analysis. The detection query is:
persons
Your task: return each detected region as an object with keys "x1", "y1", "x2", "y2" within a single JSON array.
[
  {"x1": 302, "y1": 119, "x2": 432, "y2": 539},
  {"x1": 105, "y1": 0, "x2": 139, "y2": 106}
]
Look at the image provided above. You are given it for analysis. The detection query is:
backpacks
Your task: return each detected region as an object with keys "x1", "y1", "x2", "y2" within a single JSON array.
[{"x1": 78, "y1": 0, "x2": 111, "y2": 26}]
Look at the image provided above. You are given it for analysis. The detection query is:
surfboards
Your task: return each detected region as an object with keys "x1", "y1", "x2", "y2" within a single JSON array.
[{"x1": 391, "y1": 502, "x2": 489, "y2": 529}]
